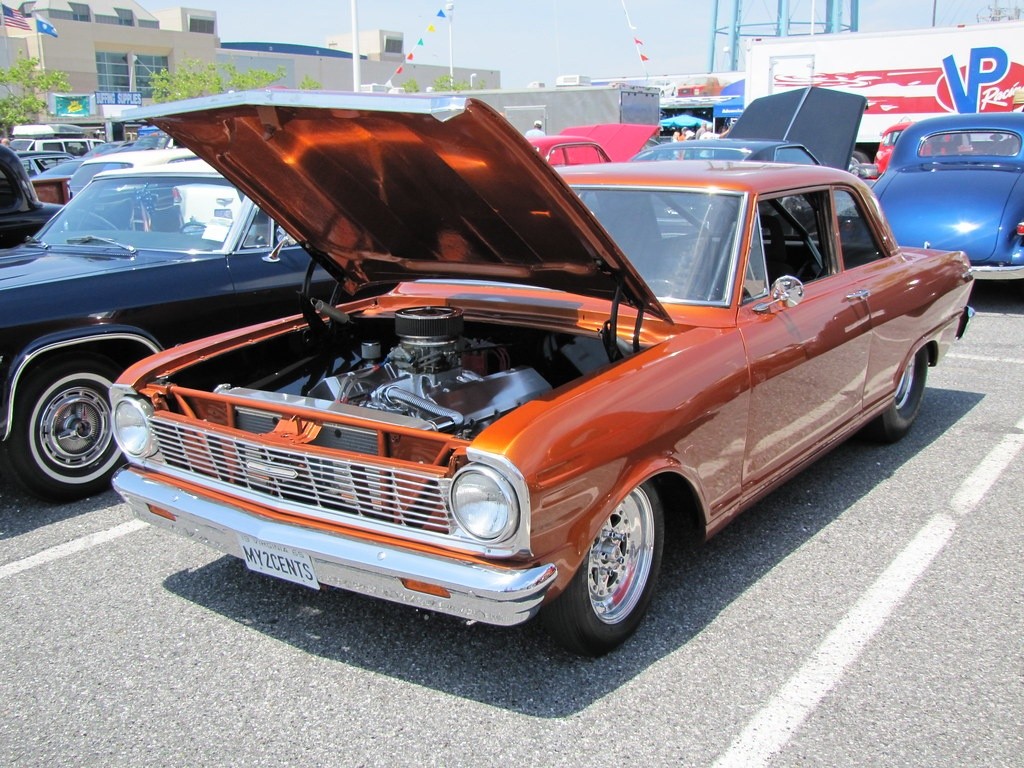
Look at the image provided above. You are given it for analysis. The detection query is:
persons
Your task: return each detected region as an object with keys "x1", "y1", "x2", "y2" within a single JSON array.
[
  {"x1": 672, "y1": 126, "x2": 697, "y2": 142},
  {"x1": 696, "y1": 121, "x2": 707, "y2": 139},
  {"x1": 2, "y1": 139, "x2": 17, "y2": 150},
  {"x1": 700, "y1": 122, "x2": 732, "y2": 140},
  {"x1": 525, "y1": 120, "x2": 546, "y2": 138}
]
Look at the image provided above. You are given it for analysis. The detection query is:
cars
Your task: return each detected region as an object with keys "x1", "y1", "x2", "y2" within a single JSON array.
[
  {"x1": 529, "y1": 123, "x2": 663, "y2": 167},
  {"x1": 0, "y1": 123, "x2": 201, "y2": 252},
  {"x1": 111, "y1": 87, "x2": 973, "y2": 656},
  {"x1": 0, "y1": 169, "x2": 338, "y2": 505},
  {"x1": 628, "y1": 85, "x2": 868, "y2": 239},
  {"x1": 869, "y1": 112, "x2": 1024, "y2": 280}
]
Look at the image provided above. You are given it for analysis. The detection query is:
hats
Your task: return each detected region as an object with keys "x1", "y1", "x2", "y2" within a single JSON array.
[
  {"x1": 685, "y1": 130, "x2": 695, "y2": 139},
  {"x1": 534, "y1": 120, "x2": 542, "y2": 128}
]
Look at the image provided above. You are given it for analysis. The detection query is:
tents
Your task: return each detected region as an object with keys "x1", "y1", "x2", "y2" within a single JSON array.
[
  {"x1": 660, "y1": 114, "x2": 712, "y2": 128},
  {"x1": 712, "y1": 96, "x2": 744, "y2": 133}
]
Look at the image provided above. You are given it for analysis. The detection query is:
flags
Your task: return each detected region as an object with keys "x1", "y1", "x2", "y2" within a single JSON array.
[
  {"x1": 1, "y1": 4, "x2": 33, "y2": 31},
  {"x1": 35, "y1": 13, "x2": 59, "y2": 37}
]
